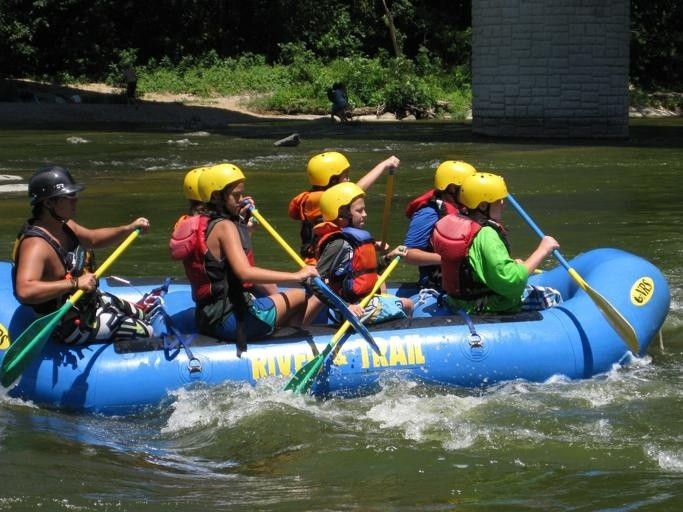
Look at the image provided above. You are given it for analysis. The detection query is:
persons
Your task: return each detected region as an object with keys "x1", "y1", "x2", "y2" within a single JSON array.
[
  {"x1": 308, "y1": 181, "x2": 415, "y2": 325},
  {"x1": 327, "y1": 80, "x2": 350, "y2": 122},
  {"x1": 402, "y1": 159, "x2": 477, "y2": 288},
  {"x1": 122, "y1": 64, "x2": 137, "y2": 101},
  {"x1": 168, "y1": 162, "x2": 308, "y2": 340},
  {"x1": 11, "y1": 165, "x2": 154, "y2": 344},
  {"x1": 184, "y1": 165, "x2": 278, "y2": 296},
  {"x1": 431, "y1": 173, "x2": 560, "y2": 313},
  {"x1": 288, "y1": 151, "x2": 399, "y2": 323}
]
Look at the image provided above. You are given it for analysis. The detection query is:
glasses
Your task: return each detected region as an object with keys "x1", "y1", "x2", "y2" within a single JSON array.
[{"x1": 59, "y1": 192, "x2": 77, "y2": 199}]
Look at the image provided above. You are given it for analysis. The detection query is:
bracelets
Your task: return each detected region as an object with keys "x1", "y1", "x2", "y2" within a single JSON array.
[{"x1": 69, "y1": 275, "x2": 79, "y2": 290}]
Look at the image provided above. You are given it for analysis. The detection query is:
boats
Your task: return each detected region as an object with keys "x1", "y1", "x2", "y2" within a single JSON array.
[{"x1": 0, "y1": 249, "x2": 668, "y2": 414}]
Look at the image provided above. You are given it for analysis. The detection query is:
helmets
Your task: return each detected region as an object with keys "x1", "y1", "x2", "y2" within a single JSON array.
[
  {"x1": 460, "y1": 172, "x2": 507, "y2": 210},
  {"x1": 28, "y1": 167, "x2": 86, "y2": 206},
  {"x1": 307, "y1": 152, "x2": 350, "y2": 187},
  {"x1": 183, "y1": 163, "x2": 246, "y2": 203},
  {"x1": 435, "y1": 160, "x2": 476, "y2": 191},
  {"x1": 320, "y1": 182, "x2": 367, "y2": 222}
]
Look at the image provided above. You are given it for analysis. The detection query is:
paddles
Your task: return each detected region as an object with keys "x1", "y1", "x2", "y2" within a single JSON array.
[
  {"x1": 240, "y1": 200, "x2": 381, "y2": 356},
  {"x1": 506, "y1": 195, "x2": 639, "y2": 355},
  {"x1": 284, "y1": 246, "x2": 407, "y2": 395},
  {"x1": 0, "y1": 228, "x2": 144, "y2": 387}
]
